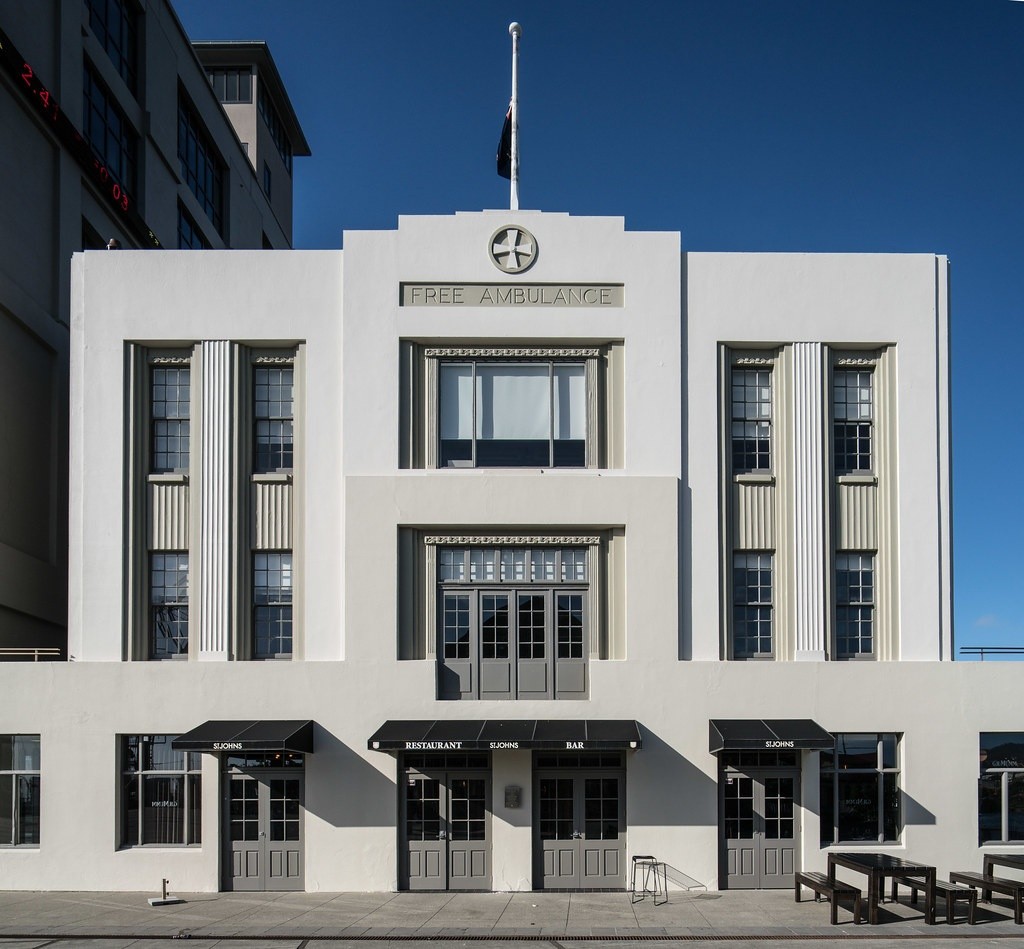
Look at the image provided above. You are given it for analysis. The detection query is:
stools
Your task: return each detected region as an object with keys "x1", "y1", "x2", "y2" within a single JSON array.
[{"x1": 629, "y1": 856, "x2": 668, "y2": 906}]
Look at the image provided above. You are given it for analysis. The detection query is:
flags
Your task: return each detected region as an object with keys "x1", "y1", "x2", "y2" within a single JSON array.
[{"x1": 497, "y1": 104, "x2": 513, "y2": 180}]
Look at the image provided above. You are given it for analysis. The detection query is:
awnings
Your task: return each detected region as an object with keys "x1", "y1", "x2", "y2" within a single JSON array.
[
  {"x1": 366, "y1": 718, "x2": 644, "y2": 754},
  {"x1": 170, "y1": 718, "x2": 316, "y2": 755},
  {"x1": 708, "y1": 717, "x2": 837, "y2": 755}
]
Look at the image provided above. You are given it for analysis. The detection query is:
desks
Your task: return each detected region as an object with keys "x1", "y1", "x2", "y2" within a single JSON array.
[
  {"x1": 981, "y1": 854, "x2": 1024, "y2": 904},
  {"x1": 827, "y1": 852, "x2": 937, "y2": 925}
]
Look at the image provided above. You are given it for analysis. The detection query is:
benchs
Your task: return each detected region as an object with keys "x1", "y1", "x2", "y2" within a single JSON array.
[
  {"x1": 950, "y1": 871, "x2": 1024, "y2": 925},
  {"x1": 891, "y1": 877, "x2": 978, "y2": 926},
  {"x1": 795, "y1": 872, "x2": 862, "y2": 925}
]
